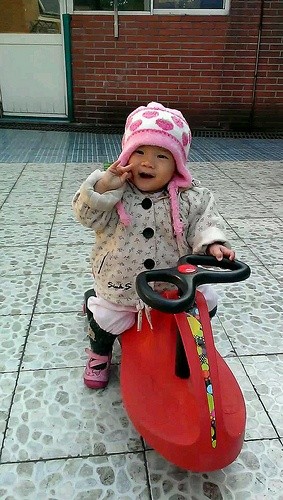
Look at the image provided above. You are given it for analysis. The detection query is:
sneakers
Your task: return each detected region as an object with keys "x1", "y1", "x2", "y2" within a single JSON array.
[{"x1": 83, "y1": 348, "x2": 112, "y2": 390}]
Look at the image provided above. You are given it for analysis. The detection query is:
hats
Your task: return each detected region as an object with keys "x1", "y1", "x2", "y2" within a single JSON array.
[{"x1": 114, "y1": 101, "x2": 192, "y2": 236}]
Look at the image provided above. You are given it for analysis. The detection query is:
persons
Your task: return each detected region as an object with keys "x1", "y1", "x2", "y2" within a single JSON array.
[{"x1": 72, "y1": 101, "x2": 236, "y2": 390}]
